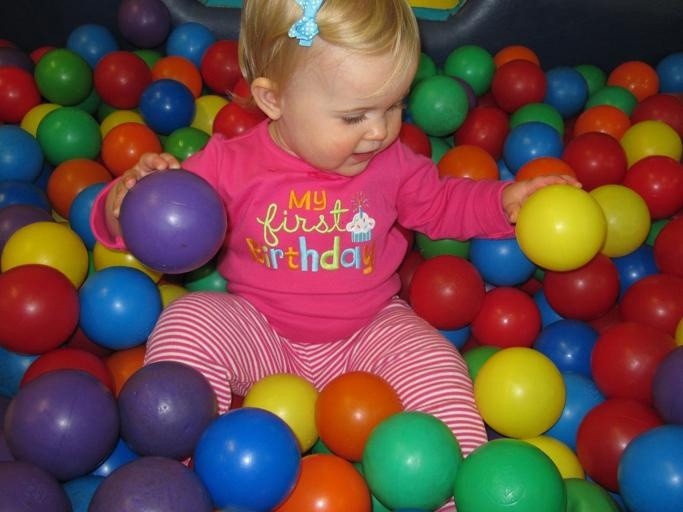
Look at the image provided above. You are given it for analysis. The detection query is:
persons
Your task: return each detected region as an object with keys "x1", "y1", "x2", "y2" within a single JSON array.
[{"x1": 0, "y1": 0, "x2": 683, "y2": 512}]
